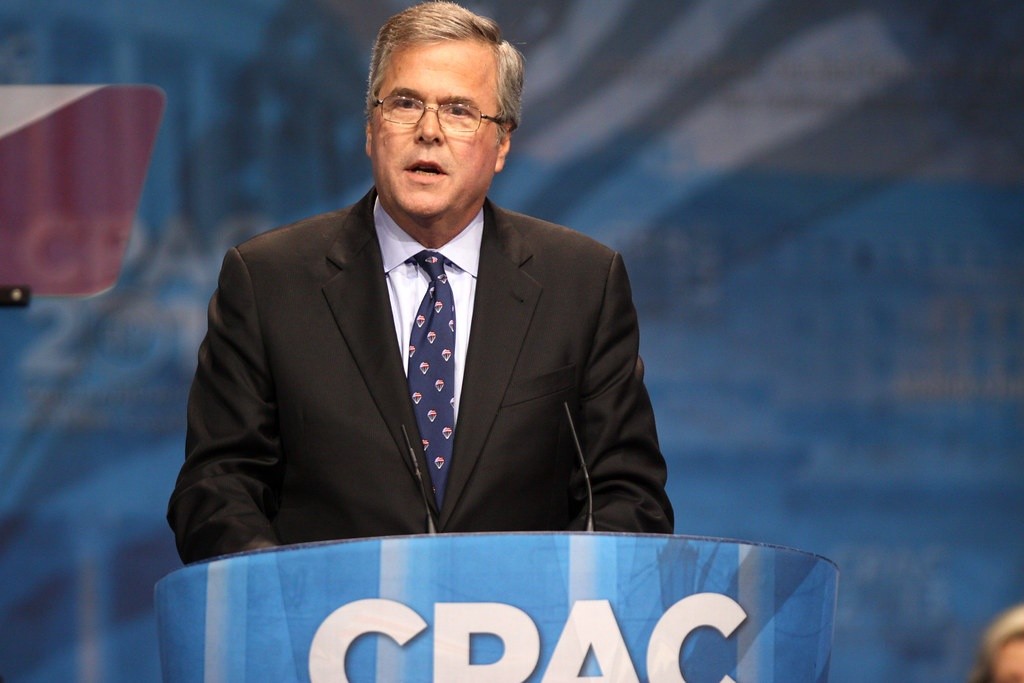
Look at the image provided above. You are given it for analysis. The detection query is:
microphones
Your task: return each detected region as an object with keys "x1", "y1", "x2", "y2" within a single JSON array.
[
  {"x1": 562, "y1": 400, "x2": 594, "y2": 532},
  {"x1": 401, "y1": 424, "x2": 435, "y2": 533}
]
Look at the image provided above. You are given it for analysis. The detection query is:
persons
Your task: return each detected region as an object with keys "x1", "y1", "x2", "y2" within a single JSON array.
[
  {"x1": 972, "y1": 599, "x2": 1023, "y2": 682},
  {"x1": 164, "y1": 0, "x2": 678, "y2": 566}
]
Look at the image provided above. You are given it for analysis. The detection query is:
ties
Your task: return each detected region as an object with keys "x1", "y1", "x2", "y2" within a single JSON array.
[{"x1": 402, "y1": 250, "x2": 464, "y2": 513}]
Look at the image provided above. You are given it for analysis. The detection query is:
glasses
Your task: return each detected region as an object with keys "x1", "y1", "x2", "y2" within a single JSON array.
[{"x1": 373, "y1": 94, "x2": 505, "y2": 132}]
